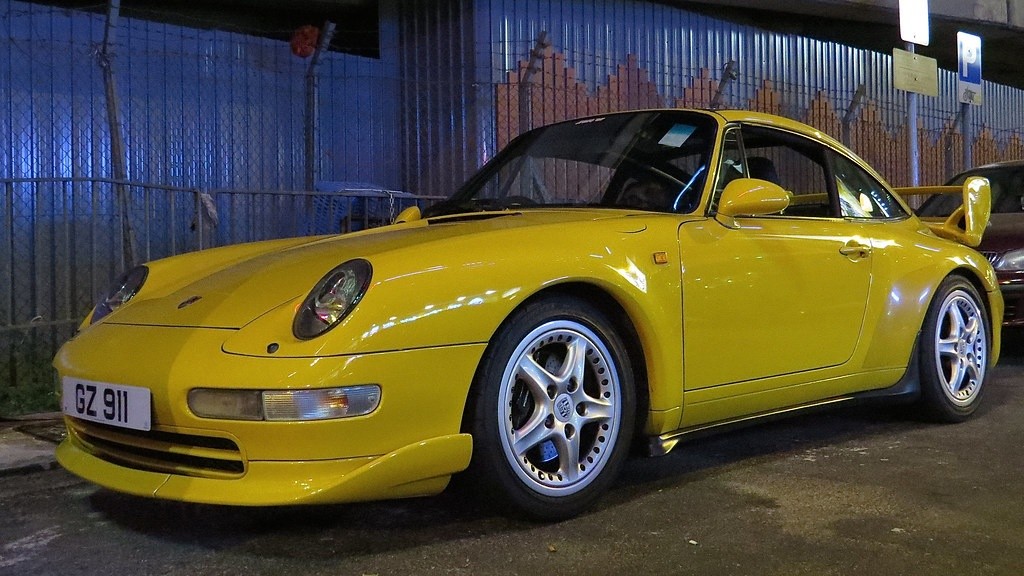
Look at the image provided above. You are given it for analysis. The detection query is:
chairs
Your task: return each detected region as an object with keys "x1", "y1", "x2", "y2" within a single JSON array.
[{"x1": 601, "y1": 156, "x2": 778, "y2": 215}]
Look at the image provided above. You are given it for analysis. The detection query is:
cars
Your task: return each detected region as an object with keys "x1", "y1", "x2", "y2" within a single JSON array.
[{"x1": 914, "y1": 160, "x2": 1024, "y2": 327}]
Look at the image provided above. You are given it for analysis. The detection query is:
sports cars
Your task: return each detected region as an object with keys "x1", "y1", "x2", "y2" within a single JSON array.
[{"x1": 51, "y1": 109, "x2": 1003, "y2": 519}]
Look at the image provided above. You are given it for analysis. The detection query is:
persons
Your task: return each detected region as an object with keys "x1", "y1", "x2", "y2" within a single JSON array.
[{"x1": 631, "y1": 175, "x2": 671, "y2": 213}]
